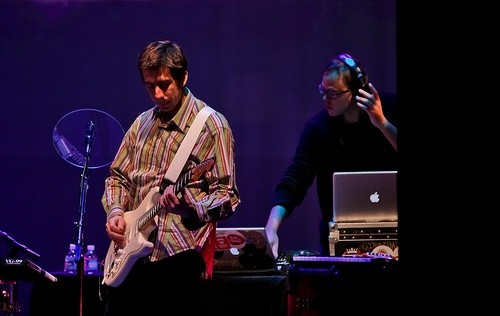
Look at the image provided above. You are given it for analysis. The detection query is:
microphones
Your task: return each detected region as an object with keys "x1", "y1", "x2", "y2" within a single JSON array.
[{"x1": 86, "y1": 121, "x2": 96, "y2": 156}]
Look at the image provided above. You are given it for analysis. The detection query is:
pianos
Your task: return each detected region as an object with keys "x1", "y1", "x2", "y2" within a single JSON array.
[{"x1": 287, "y1": 250, "x2": 400, "y2": 269}]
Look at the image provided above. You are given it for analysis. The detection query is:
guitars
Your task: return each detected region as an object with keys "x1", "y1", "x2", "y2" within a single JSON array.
[{"x1": 101, "y1": 156, "x2": 218, "y2": 289}]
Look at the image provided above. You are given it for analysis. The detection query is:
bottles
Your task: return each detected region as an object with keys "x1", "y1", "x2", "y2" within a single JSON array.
[
  {"x1": 83, "y1": 245, "x2": 98, "y2": 276},
  {"x1": 64, "y1": 244, "x2": 78, "y2": 275}
]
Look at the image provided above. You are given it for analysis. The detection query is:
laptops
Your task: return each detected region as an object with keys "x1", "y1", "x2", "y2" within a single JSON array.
[
  {"x1": 332, "y1": 171, "x2": 399, "y2": 222},
  {"x1": 212, "y1": 228, "x2": 286, "y2": 276}
]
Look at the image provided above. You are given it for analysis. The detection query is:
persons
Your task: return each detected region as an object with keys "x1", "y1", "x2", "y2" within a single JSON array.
[
  {"x1": 100, "y1": 40, "x2": 241, "y2": 316},
  {"x1": 264, "y1": 53, "x2": 397, "y2": 259}
]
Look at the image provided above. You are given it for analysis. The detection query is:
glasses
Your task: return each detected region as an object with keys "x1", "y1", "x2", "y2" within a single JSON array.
[{"x1": 319, "y1": 85, "x2": 350, "y2": 97}]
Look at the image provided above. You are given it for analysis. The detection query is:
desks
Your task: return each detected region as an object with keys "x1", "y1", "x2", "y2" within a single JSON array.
[{"x1": 30, "y1": 271, "x2": 318, "y2": 316}]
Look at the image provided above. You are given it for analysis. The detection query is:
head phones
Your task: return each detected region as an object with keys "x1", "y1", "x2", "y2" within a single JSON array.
[{"x1": 337, "y1": 53, "x2": 370, "y2": 99}]
